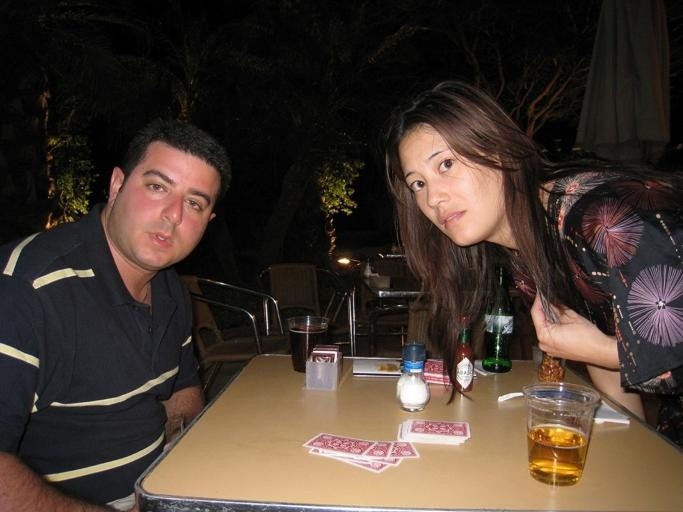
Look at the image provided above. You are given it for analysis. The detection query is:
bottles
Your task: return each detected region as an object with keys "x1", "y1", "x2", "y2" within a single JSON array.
[
  {"x1": 395, "y1": 342, "x2": 431, "y2": 412},
  {"x1": 481, "y1": 265, "x2": 515, "y2": 372},
  {"x1": 451, "y1": 314, "x2": 474, "y2": 392}
]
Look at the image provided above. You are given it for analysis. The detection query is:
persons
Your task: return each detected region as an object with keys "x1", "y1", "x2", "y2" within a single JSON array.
[
  {"x1": 0, "y1": 118, "x2": 236, "y2": 510},
  {"x1": 378, "y1": 78, "x2": 683, "y2": 452}
]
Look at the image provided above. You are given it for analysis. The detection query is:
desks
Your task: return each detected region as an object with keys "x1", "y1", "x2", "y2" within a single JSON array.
[
  {"x1": 134, "y1": 353, "x2": 683, "y2": 512},
  {"x1": 355, "y1": 258, "x2": 420, "y2": 356}
]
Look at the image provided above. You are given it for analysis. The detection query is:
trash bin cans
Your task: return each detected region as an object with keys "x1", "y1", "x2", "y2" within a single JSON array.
[{"x1": 357, "y1": 334, "x2": 372, "y2": 357}]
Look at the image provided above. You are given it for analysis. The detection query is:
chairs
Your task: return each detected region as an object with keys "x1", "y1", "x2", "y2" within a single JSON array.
[
  {"x1": 257, "y1": 263, "x2": 355, "y2": 355},
  {"x1": 180, "y1": 275, "x2": 291, "y2": 390}
]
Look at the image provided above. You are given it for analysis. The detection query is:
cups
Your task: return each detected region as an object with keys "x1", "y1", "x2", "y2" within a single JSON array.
[
  {"x1": 521, "y1": 381, "x2": 602, "y2": 487},
  {"x1": 287, "y1": 315, "x2": 329, "y2": 373}
]
